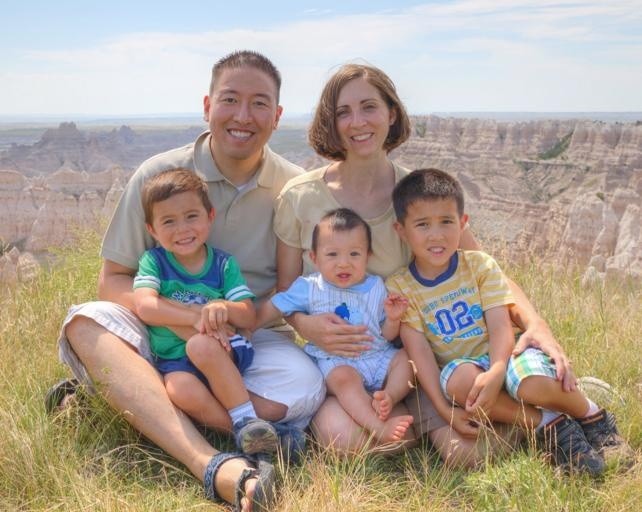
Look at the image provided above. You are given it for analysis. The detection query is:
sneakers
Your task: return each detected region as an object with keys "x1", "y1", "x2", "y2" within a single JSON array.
[
  {"x1": 233, "y1": 418, "x2": 311, "y2": 464},
  {"x1": 540, "y1": 408, "x2": 634, "y2": 477}
]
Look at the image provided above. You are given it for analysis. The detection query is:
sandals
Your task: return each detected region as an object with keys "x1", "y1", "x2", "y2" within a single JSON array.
[{"x1": 205, "y1": 451, "x2": 280, "y2": 512}]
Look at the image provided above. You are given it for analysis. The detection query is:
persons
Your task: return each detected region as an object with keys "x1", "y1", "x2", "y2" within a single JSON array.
[
  {"x1": 45, "y1": 50, "x2": 327, "y2": 512},
  {"x1": 240, "y1": 206, "x2": 418, "y2": 446},
  {"x1": 272, "y1": 61, "x2": 526, "y2": 472},
  {"x1": 131, "y1": 168, "x2": 280, "y2": 459},
  {"x1": 386, "y1": 167, "x2": 637, "y2": 479}
]
